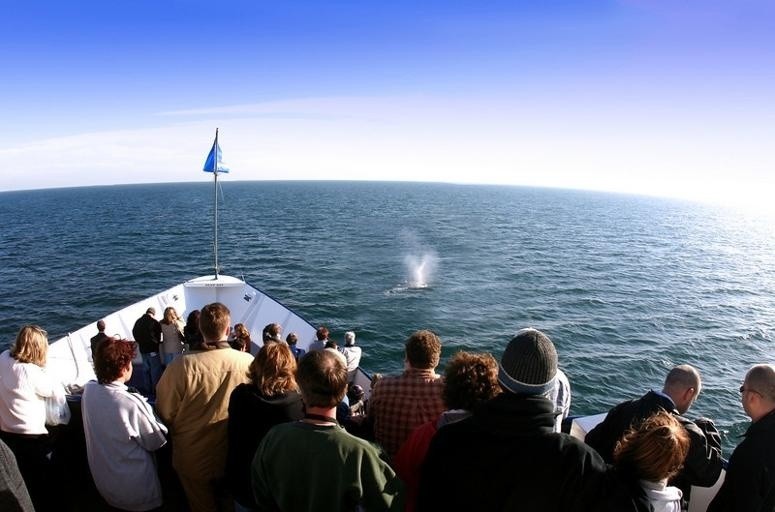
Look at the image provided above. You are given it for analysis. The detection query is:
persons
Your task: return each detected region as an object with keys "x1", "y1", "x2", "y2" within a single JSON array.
[
  {"x1": 250, "y1": 349, "x2": 407, "y2": 511},
  {"x1": 132, "y1": 306, "x2": 164, "y2": 398},
  {"x1": 0, "y1": 324, "x2": 72, "y2": 511},
  {"x1": 340, "y1": 330, "x2": 361, "y2": 385},
  {"x1": 585, "y1": 364, "x2": 723, "y2": 512},
  {"x1": 706, "y1": 363, "x2": 775, "y2": 512},
  {"x1": 158, "y1": 306, "x2": 184, "y2": 367},
  {"x1": 420, "y1": 328, "x2": 610, "y2": 511},
  {"x1": 155, "y1": 301, "x2": 255, "y2": 510},
  {"x1": 309, "y1": 328, "x2": 330, "y2": 349},
  {"x1": 390, "y1": 350, "x2": 500, "y2": 511},
  {"x1": 283, "y1": 333, "x2": 304, "y2": 359},
  {"x1": 89, "y1": 321, "x2": 110, "y2": 356},
  {"x1": 228, "y1": 340, "x2": 305, "y2": 511},
  {"x1": 81, "y1": 337, "x2": 169, "y2": 512},
  {"x1": 185, "y1": 309, "x2": 205, "y2": 351},
  {"x1": 230, "y1": 322, "x2": 257, "y2": 349},
  {"x1": 611, "y1": 407, "x2": 691, "y2": 512},
  {"x1": 365, "y1": 329, "x2": 446, "y2": 458}
]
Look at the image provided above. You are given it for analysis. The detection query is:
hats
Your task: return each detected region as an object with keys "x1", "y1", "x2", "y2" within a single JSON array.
[{"x1": 498, "y1": 329, "x2": 558, "y2": 397}]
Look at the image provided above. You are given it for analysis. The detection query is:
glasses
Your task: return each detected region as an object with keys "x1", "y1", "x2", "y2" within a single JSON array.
[{"x1": 739, "y1": 386, "x2": 763, "y2": 398}]
